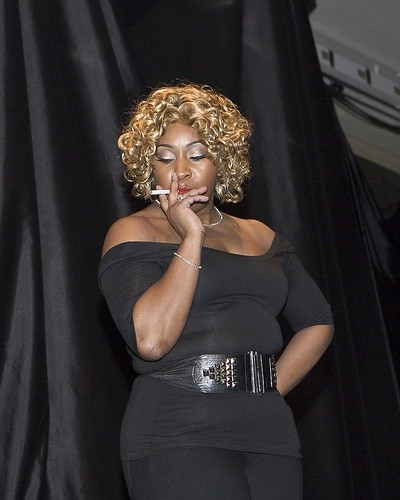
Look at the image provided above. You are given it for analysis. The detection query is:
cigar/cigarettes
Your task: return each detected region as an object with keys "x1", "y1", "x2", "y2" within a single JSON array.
[{"x1": 149, "y1": 190, "x2": 179, "y2": 196}]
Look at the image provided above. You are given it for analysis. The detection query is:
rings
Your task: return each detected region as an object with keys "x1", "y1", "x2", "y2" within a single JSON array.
[{"x1": 176, "y1": 194, "x2": 187, "y2": 202}]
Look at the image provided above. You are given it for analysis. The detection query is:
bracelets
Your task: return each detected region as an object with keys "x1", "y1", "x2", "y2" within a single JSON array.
[{"x1": 172, "y1": 251, "x2": 203, "y2": 270}]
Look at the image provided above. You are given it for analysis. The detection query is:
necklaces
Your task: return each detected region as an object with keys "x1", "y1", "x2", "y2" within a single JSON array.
[{"x1": 155, "y1": 198, "x2": 223, "y2": 226}]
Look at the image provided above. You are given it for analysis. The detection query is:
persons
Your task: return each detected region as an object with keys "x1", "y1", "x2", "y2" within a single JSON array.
[{"x1": 97, "y1": 84, "x2": 335, "y2": 500}]
